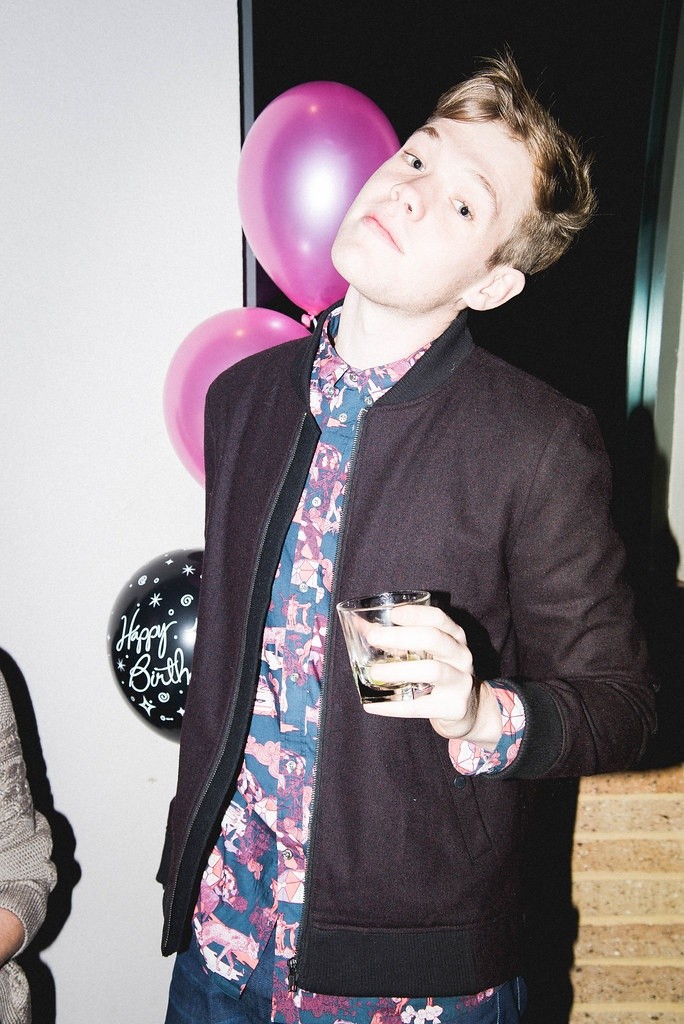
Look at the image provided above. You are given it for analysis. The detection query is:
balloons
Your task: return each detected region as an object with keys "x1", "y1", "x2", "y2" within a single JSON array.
[
  {"x1": 162, "y1": 307, "x2": 313, "y2": 490},
  {"x1": 107, "y1": 549, "x2": 205, "y2": 731},
  {"x1": 237, "y1": 81, "x2": 401, "y2": 328}
]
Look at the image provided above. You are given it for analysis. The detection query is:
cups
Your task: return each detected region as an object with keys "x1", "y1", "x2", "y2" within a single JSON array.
[{"x1": 337, "y1": 590, "x2": 431, "y2": 705}]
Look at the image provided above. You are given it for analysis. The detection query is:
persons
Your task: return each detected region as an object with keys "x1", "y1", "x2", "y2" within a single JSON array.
[
  {"x1": 0, "y1": 671, "x2": 58, "y2": 1024},
  {"x1": 156, "y1": 43, "x2": 659, "y2": 1024}
]
